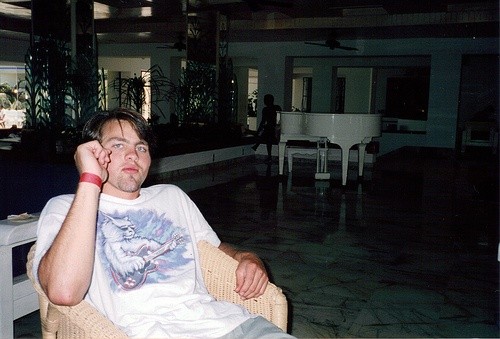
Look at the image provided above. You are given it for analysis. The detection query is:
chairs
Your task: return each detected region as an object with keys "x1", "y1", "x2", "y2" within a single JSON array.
[{"x1": 26, "y1": 240, "x2": 288, "y2": 339}]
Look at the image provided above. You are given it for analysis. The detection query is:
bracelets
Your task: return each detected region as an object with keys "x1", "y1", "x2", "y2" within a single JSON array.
[{"x1": 77, "y1": 173, "x2": 105, "y2": 190}]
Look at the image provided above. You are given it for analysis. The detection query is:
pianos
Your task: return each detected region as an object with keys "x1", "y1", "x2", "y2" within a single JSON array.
[{"x1": 276, "y1": 111, "x2": 384, "y2": 186}]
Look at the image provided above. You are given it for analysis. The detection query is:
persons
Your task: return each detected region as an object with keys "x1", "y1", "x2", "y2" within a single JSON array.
[
  {"x1": 32, "y1": 107, "x2": 301, "y2": 339},
  {"x1": 252, "y1": 94, "x2": 282, "y2": 155}
]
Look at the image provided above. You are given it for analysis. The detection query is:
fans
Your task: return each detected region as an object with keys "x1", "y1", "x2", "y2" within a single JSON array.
[
  {"x1": 304, "y1": 33, "x2": 359, "y2": 51},
  {"x1": 156, "y1": 34, "x2": 186, "y2": 52}
]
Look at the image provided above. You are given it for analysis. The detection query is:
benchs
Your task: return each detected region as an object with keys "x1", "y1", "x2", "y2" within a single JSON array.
[{"x1": 286, "y1": 147, "x2": 325, "y2": 172}]
